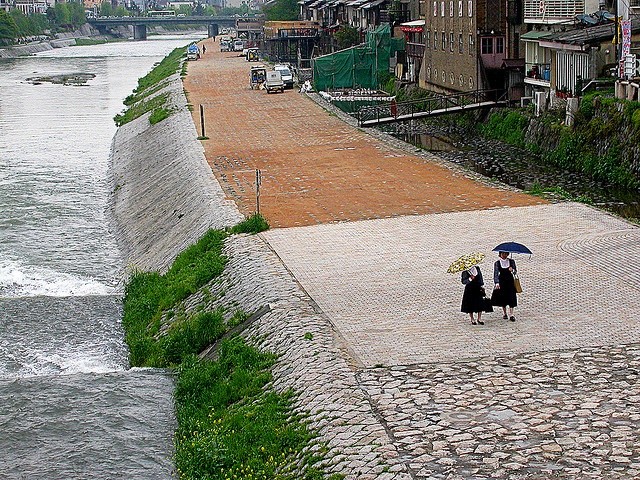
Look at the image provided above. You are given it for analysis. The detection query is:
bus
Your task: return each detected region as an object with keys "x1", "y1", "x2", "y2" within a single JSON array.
[{"x1": 151, "y1": 11, "x2": 176, "y2": 19}]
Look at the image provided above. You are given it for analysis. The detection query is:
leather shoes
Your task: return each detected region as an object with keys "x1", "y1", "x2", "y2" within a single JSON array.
[
  {"x1": 503, "y1": 315, "x2": 508, "y2": 319},
  {"x1": 471, "y1": 320, "x2": 476, "y2": 325},
  {"x1": 477, "y1": 319, "x2": 484, "y2": 325},
  {"x1": 510, "y1": 316, "x2": 515, "y2": 322}
]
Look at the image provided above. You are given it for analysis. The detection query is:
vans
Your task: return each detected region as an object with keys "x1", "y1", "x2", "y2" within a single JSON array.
[
  {"x1": 272, "y1": 66, "x2": 295, "y2": 89},
  {"x1": 188, "y1": 43, "x2": 201, "y2": 61},
  {"x1": 177, "y1": 14, "x2": 186, "y2": 18},
  {"x1": 220, "y1": 37, "x2": 233, "y2": 52},
  {"x1": 222, "y1": 29, "x2": 228, "y2": 35},
  {"x1": 234, "y1": 41, "x2": 243, "y2": 52},
  {"x1": 248, "y1": 48, "x2": 259, "y2": 62},
  {"x1": 250, "y1": 66, "x2": 267, "y2": 90}
]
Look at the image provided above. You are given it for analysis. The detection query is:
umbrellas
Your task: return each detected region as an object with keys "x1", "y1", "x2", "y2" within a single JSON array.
[
  {"x1": 491, "y1": 242, "x2": 533, "y2": 259},
  {"x1": 446, "y1": 251, "x2": 487, "y2": 276}
]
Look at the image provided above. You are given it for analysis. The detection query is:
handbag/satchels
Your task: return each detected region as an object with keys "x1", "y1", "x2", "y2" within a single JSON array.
[
  {"x1": 513, "y1": 272, "x2": 523, "y2": 293},
  {"x1": 484, "y1": 297, "x2": 493, "y2": 313},
  {"x1": 480, "y1": 287, "x2": 485, "y2": 297}
]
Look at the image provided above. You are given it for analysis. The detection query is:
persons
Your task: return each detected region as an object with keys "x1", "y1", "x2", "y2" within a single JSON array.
[
  {"x1": 391, "y1": 98, "x2": 399, "y2": 118},
  {"x1": 490, "y1": 251, "x2": 517, "y2": 321},
  {"x1": 461, "y1": 266, "x2": 484, "y2": 325}
]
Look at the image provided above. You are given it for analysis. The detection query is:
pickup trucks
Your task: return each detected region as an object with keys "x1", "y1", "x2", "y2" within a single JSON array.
[{"x1": 263, "y1": 71, "x2": 286, "y2": 94}]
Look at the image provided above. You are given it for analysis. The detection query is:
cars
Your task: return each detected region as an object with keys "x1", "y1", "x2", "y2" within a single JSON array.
[{"x1": 240, "y1": 49, "x2": 248, "y2": 57}]
